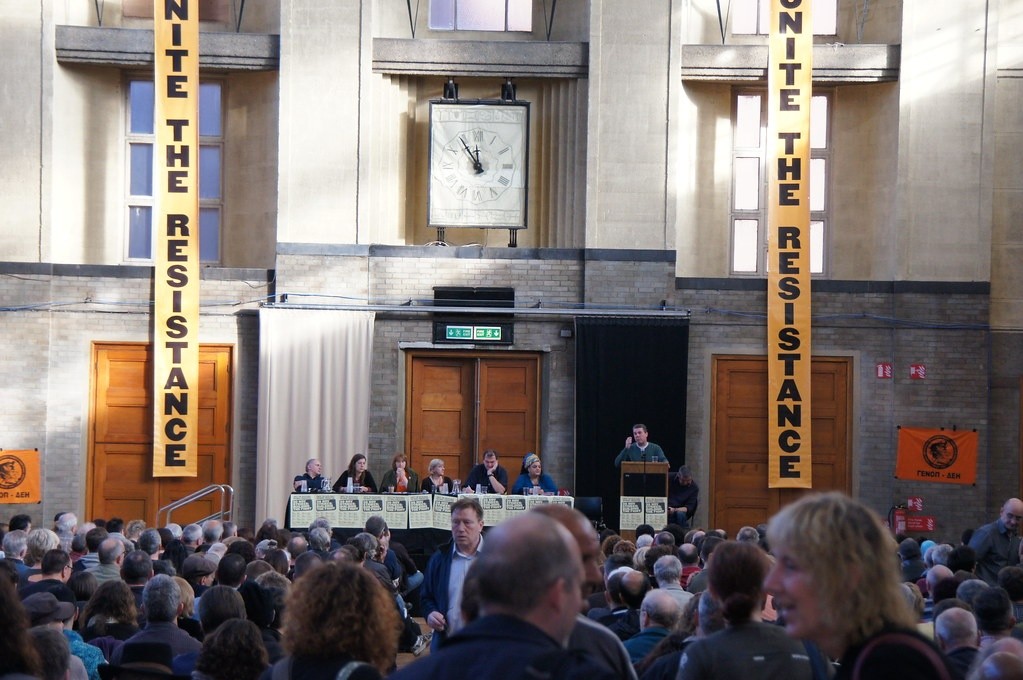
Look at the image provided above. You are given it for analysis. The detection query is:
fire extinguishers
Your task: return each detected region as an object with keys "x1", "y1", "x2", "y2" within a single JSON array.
[{"x1": 889, "y1": 503, "x2": 908, "y2": 535}]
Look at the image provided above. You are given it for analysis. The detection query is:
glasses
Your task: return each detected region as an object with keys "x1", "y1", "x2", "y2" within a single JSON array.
[{"x1": 65, "y1": 564, "x2": 75, "y2": 573}]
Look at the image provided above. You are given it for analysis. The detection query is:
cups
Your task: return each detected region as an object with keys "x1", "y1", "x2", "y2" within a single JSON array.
[
  {"x1": 346, "y1": 477, "x2": 353, "y2": 493},
  {"x1": 522, "y1": 486, "x2": 530, "y2": 495},
  {"x1": 354, "y1": 483, "x2": 361, "y2": 493},
  {"x1": 475, "y1": 484, "x2": 482, "y2": 494},
  {"x1": 441, "y1": 483, "x2": 449, "y2": 494},
  {"x1": 532, "y1": 486, "x2": 541, "y2": 495},
  {"x1": 432, "y1": 484, "x2": 438, "y2": 493},
  {"x1": 482, "y1": 486, "x2": 488, "y2": 494},
  {"x1": 301, "y1": 480, "x2": 308, "y2": 492}
]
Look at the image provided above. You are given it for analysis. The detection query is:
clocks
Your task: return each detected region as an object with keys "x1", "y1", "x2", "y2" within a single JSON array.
[{"x1": 427, "y1": 98, "x2": 530, "y2": 229}]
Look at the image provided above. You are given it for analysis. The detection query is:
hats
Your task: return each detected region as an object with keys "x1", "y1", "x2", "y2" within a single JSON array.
[
  {"x1": 21, "y1": 591, "x2": 75, "y2": 627},
  {"x1": 181, "y1": 552, "x2": 220, "y2": 578}
]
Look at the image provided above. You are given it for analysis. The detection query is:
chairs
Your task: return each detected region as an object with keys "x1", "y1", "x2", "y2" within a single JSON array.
[{"x1": 573, "y1": 495, "x2": 607, "y2": 533}]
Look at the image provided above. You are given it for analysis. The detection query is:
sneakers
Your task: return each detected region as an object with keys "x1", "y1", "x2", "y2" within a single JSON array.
[{"x1": 410, "y1": 631, "x2": 433, "y2": 657}]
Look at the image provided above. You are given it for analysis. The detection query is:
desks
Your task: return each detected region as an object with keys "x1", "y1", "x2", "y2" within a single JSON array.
[
  {"x1": 434, "y1": 492, "x2": 573, "y2": 540},
  {"x1": 285, "y1": 493, "x2": 433, "y2": 540}
]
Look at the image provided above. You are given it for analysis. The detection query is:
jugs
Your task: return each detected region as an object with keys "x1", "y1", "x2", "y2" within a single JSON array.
[{"x1": 320, "y1": 477, "x2": 333, "y2": 493}]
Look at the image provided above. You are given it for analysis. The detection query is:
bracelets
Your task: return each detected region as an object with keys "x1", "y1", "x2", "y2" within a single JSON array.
[{"x1": 488, "y1": 474, "x2": 494, "y2": 477}]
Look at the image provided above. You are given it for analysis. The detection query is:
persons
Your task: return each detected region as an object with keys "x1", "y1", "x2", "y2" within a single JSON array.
[
  {"x1": 292, "y1": 459, "x2": 325, "y2": 494},
  {"x1": 666, "y1": 465, "x2": 700, "y2": 530},
  {"x1": 0, "y1": 494, "x2": 1023, "y2": 678},
  {"x1": 461, "y1": 449, "x2": 508, "y2": 495},
  {"x1": 511, "y1": 452, "x2": 559, "y2": 495},
  {"x1": 378, "y1": 454, "x2": 420, "y2": 494},
  {"x1": 613, "y1": 422, "x2": 671, "y2": 468},
  {"x1": 420, "y1": 459, "x2": 453, "y2": 493},
  {"x1": 331, "y1": 454, "x2": 378, "y2": 494}
]
